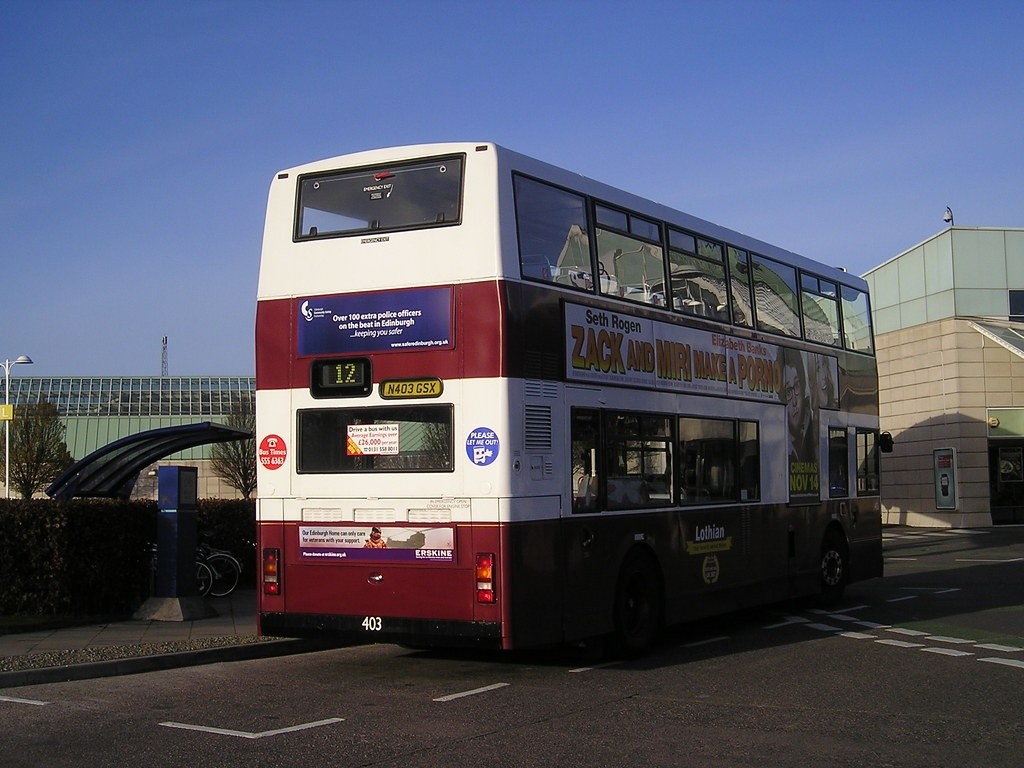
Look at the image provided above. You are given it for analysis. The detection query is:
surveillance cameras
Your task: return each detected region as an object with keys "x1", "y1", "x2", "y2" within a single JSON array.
[{"x1": 944, "y1": 211, "x2": 952, "y2": 223}]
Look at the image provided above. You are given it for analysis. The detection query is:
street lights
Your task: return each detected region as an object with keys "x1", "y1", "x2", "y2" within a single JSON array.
[{"x1": 0, "y1": 354, "x2": 34, "y2": 498}]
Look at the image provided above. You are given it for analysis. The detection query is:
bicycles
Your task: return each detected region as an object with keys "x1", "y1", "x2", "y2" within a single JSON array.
[{"x1": 136, "y1": 532, "x2": 241, "y2": 600}]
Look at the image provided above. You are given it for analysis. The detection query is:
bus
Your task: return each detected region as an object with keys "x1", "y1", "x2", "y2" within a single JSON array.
[{"x1": 251, "y1": 140, "x2": 895, "y2": 654}]
